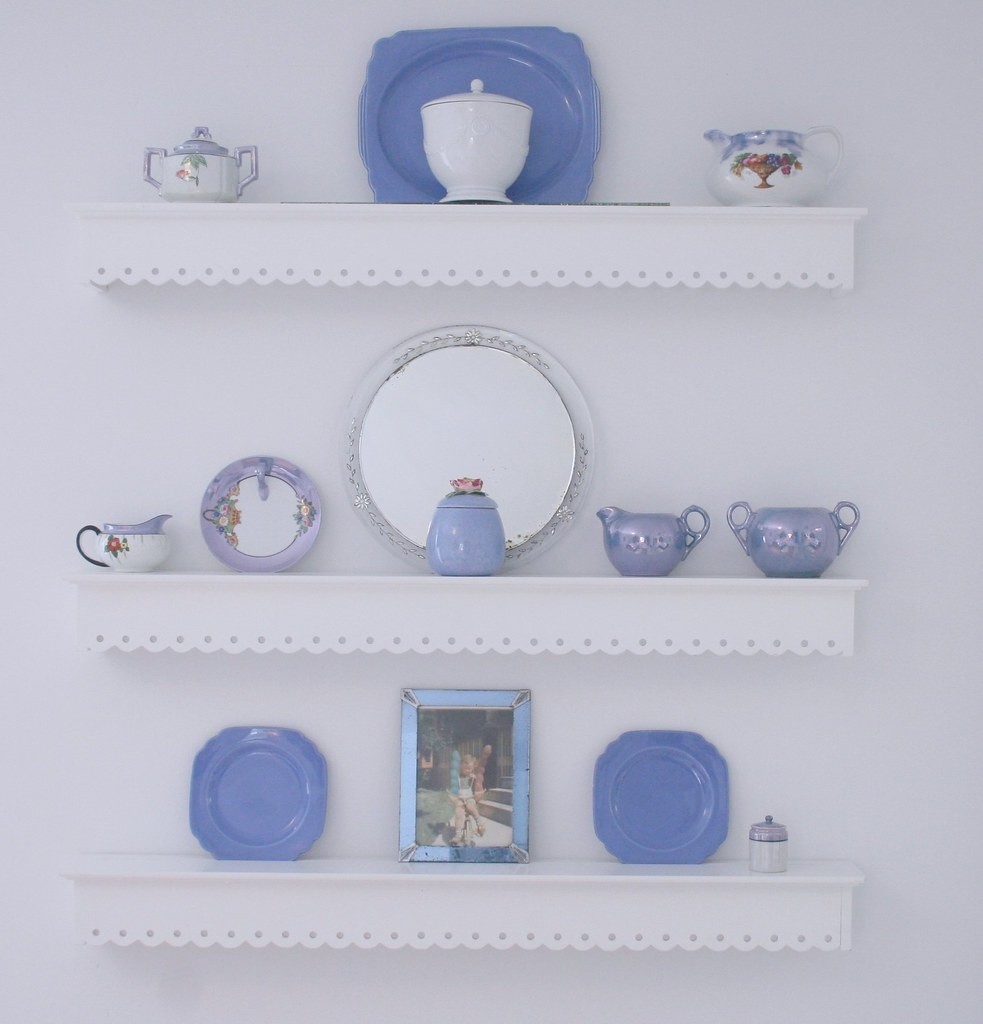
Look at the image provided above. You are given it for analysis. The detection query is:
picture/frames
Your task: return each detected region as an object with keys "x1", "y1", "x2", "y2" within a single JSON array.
[{"x1": 401, "y1": 688, "x2": 532, "y2": 864}]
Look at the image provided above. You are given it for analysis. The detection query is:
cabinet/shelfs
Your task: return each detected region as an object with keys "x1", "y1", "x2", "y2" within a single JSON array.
[{"x1": 56, "y1": 199, "x2": 870, "y2": 950}]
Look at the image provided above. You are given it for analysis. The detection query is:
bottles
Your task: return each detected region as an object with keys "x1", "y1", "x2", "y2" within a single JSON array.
[{"x1": 141, "y1": 127, "x2": 258, "y2": 203}]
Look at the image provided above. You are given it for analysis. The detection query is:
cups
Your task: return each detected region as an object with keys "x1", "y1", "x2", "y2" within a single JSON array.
[
  {"x1": 426, "y1": 493, "x2": 506, "y2": 576},
  {"x1": 749, "y1": 815, "x2": 788, "y2": 873},
  {"x1": 418, "y1": 78, "x2": 534, "y2": 203},
  {"x1": 726, "y1": 501, "x2": 860, "y2": 578}
]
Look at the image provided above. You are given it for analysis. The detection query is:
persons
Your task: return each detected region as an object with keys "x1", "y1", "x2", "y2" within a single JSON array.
[
  {"x1": 475, "y1": 744, "x2": 497, "y2": 791},
  {"x1": 447, "y1": 753, "x2": 487, "y2": 846}
]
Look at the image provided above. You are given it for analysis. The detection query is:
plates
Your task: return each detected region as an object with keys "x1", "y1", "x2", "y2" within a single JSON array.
[
  {"x1": 189, "y1": 727, "x2": 328, "y2": 861},
  {"x1": 593, "y1": 729, "x2": 732, "y2": 865},
  {"x1": 200, "y1": 456, "x2": 320, "y2": 573},
  {"x1": 358, "y1": 27, "x2": 601, "y2": 203}
]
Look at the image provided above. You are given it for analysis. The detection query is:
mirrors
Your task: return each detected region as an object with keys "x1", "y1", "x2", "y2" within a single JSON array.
[
  {"x1": 340, "y1": 325, "x2": 594, "y2": 574},
  {"x1": 199, "y1": 454, "x2": 322, "y2": 572}
]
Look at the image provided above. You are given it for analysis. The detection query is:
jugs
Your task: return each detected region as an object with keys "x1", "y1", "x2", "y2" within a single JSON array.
[
  {"x1": 596, "y1": 504, "x2": 711, "y2": 577},
  {"x1": 76, "y1": 514, "x2": 173, "y2": 573},
  {"x1": 700, "y1": 125, "x2": 844, "y2": 205}
]
